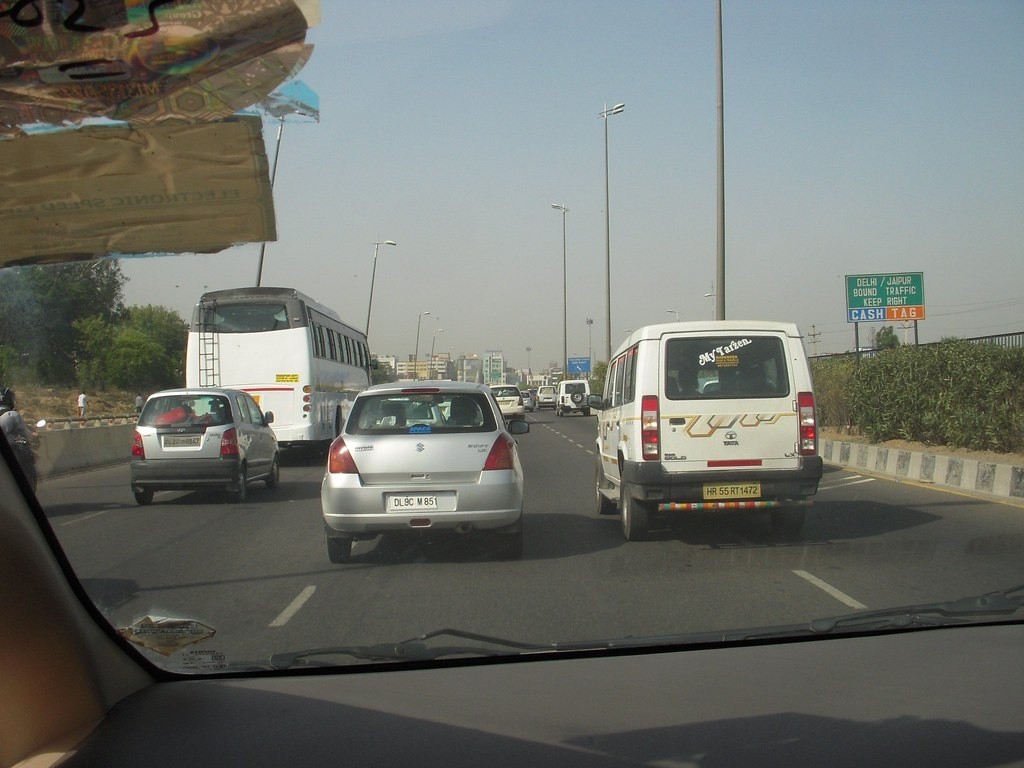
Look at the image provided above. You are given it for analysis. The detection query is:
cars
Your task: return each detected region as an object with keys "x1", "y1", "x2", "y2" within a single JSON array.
[
  {"x1": 131, "y1": 386, "x2": 281, "y2": 507},
  {"x1": 320, "y1": 380, "x2": 530, "y2": 565},
  {"x1": 378, "y1": 381, "x2": 557, "y2": 420}
]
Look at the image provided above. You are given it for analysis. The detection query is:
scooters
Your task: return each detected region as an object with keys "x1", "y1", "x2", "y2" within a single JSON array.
[{"x1": 8, "y1": 420, "x2": 47, "y2": 492}]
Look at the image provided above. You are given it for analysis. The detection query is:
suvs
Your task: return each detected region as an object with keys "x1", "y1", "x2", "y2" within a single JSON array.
[{"x1": 553, "y1": 379, "x2": 591, "y2": 417}]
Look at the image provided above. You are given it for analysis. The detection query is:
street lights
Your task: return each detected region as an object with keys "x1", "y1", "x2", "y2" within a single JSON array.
[
  {"x1": 597, "y1": 102, "x2": 630, "y2": 364},
  {"x1": 428, "y1": 327, "x2": 444, "y2": 379},
  {"x1": 525, "y1": 346, "x2": 532, "y2": 385},
  {"x1": 412, "y1": 312, "x2": 431, "y2": 381},
  {"x1": 666, "y1": 310, "x2": 680, "y2": 322},
  {"x1": 366, "y1": 240, "x2": 397, "y2": 336},
  {"x1": 550, "y1": 202, "x2": 569, "y2": 381},
  {"x1": 585, "y1": 317, "x2": 595, "y2": 379},
  {"x1": 254, "y1": 108, "x2": 309, "y2": 287}
]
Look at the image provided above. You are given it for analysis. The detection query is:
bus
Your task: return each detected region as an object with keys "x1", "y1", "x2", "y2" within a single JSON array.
[{"x1": 183, "y1": 286, "x2": 373, "y2": 450}]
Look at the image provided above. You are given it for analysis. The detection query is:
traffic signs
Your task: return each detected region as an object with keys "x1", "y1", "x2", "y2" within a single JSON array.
[{"x1": 845, "y1": 272, "x2": 925, "y2": 321}]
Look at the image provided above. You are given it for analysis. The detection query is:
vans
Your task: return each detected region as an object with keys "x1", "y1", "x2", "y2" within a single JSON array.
[{"x1": 585, "y1": 319, "x2": 825, "y2": 542}]
